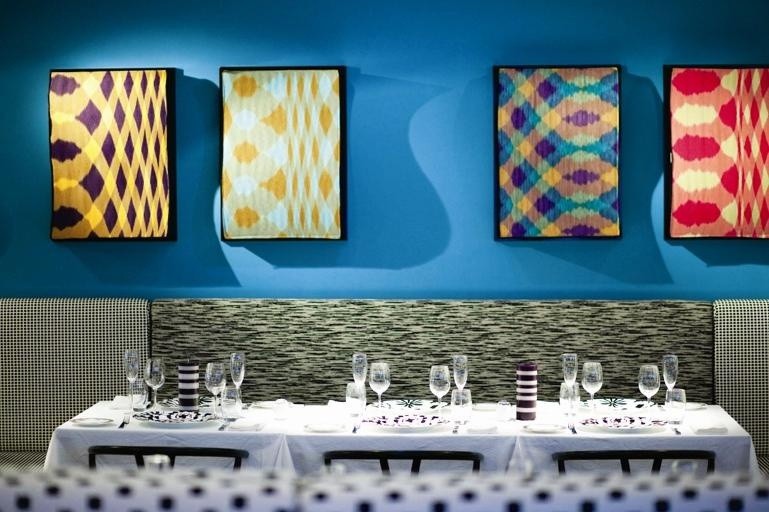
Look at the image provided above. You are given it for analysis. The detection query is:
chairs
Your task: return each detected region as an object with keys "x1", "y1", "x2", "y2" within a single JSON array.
[
  {"x1": 89, "y1": 445, "x2": 249, "y2": 477},
  {"x1": 550, "y1": 450, "x2": 717, "y2": 476},
  {"x1": 323, "y1": 449, "x2": 484, "y2": 476}
]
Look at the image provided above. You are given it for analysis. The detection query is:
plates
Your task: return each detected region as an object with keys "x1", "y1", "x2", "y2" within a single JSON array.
[
  {"x1": 524, "y1": 421, "x2": 568, "y2": 434},
  {"x1": 577, "y1": 415, "x2": 667, "y2": 431},
  {"x1": 156, "y1": 394, "x2": 237, "y2": 411},
  {"x1": 582, "y1": 398, "x2": 665, "y2": 415},
  {"x1": 303, "y1": 422, "x2": 347, "y2": 434},
  {"x1": 361, "y1": 412, "x2": 449, "y2": 430},
  {"x1": 71, "y1": 416, "x2": 114, "y2": 427},
  {"x1": 372, "y1": 397, "x2": 449, "y2": 413},
  {"x1": 132, "y1": 408, "x2": 216, "y2": 425}
]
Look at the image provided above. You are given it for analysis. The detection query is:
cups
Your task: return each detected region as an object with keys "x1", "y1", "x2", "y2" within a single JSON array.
[
  {"x1": 128, "y1": 378, "x2": 150, "y2": 412},
  {"x1": 495, "y1": 400, "x2": 513, "y2": 421},
  {"x1": 666, "y1": 387, "x2": 687, "y2": 424},
  {"x1": 448, "y1": 389, "x2": 475, "y2": 424},
  {"x1": 345, "y1": 382, "x2": 367, "y2": 418},
  {"x1": 220, "y1": 387, "x2": 244, "y2": 418},
  {"x1": 351, "y1": 351, "x2": 368, "y2": 386},
  {"x1": 558, "y1": 379, "x2": 581, "y2": 417}
]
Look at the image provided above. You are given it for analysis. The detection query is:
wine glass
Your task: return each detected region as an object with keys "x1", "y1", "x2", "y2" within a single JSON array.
[
  {"x1": 229, "y1": 351, "x2": 248, "y2": 411},
  {"x1": 143, "y1": 356, "x2": 167, "y2": 410},
  {"x1": 561, "y1": 353, "x2": 604, "y2": 415},
  {"x1": 450, "y1": 353, "x2": 471, "y2": 406},
  {"x1": 637, "y1": 354, "x2": 679, "y2": 410},
  {"x1": 122, "y1": 346, "x2": 141, "y2": 415},
  {"x1": 368, "y1": 358, "x2": 393, "y2": 414},
  {"x1": 204, "y1": 359, "x2": 226, "y2": 420},
  {"x1": 428, "y1": 361, "x2": 451, "y2": 416}
]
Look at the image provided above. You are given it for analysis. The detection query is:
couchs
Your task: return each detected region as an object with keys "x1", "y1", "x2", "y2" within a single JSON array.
[
  {"x1": 152, "y1": 297, "x2": 712, "y2": 402},
  {"x1": 2, "y1": 296, "x2": 148, "y2": 475},
  {"x1": 713, "y1": 300, "x2": 769, "y2": 475}
]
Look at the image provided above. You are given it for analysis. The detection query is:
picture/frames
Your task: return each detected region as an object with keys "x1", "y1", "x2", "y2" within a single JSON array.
[
  {"x1": 663, "y1": 64, "x2": 767, "y2": 239},
  {"x1": 494, "y1": 65, "x2": 622, "y2": 238},
  {"x1": 219, "y1": 67, "x2": 345, "y2": 240},
  {"x1": 48, "y1": 67, "x2": 177, "y2": 242}
]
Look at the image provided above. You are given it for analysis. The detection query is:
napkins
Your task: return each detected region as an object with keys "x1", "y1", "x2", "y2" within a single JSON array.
[
  {"x1": 526, "y1": 423, "x2": 555, "y2": 433},
  {"x1": 233, "y1": 416, "x2": 262, "y2": 430},
  {"x1": 692, "y1": 419, "x2": 726, "y2": 433},
  {"x1": 308, "y1": 419, "x2": 341, "y2": 432}
]
